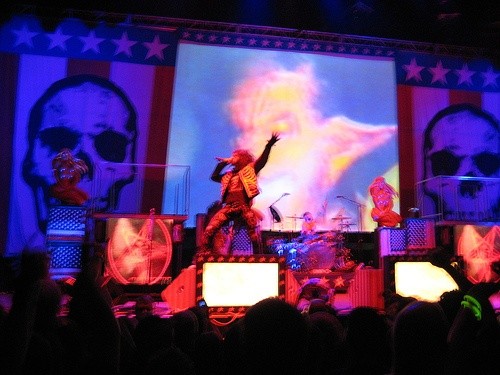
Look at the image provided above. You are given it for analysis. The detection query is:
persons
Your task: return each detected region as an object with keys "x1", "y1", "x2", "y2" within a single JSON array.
[
  {"x1": 47, "y1": 147, "x2": 91, "y2": 207},
  {"x1": 302, "y1": 212, "x2": 316, "y2": 236},
  {"x1": 368, "y1": 176, "x2": 402, "y2": 227},
  {"x1": 195, "y1": 131, "x2": 281, "y2": 256},
  {"x1": 221, "y1": 56, "x2": 398, "y2": 229},
  {"x1": 0, "y1": 247, "x2": 500, "y2": 375}
]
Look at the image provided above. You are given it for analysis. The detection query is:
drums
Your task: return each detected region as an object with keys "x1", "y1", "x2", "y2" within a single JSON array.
[{"x1": 273, "y1": 229, "x2": 343, "y2": 273}]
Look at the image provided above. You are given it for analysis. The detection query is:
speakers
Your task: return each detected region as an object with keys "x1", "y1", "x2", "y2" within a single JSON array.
[
  {"x1": 195, "y1": 253, "x2": 286, "y2": 314},
  {"x1": 383, "y1": 255, "x2": 466, "y2": 312}
]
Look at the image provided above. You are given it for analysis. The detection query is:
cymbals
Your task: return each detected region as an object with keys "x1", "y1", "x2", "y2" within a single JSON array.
[
  {"x1": 339, "y1": 223, "x2": 357, "y2": 225},
  {"x1": 331, "y1": 216, "x2": 351, "y2": 219},
  {"x1": 287, "y1": 216, "x2": 304, "y2": 219},
  {"x1": 270, "y1": 207, "x2": 281, "y2": 222}
]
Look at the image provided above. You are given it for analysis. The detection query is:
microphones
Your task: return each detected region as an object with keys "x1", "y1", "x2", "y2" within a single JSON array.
[{"x1": 216, "y1": 156, "x2": 237, "y2": 164}]
